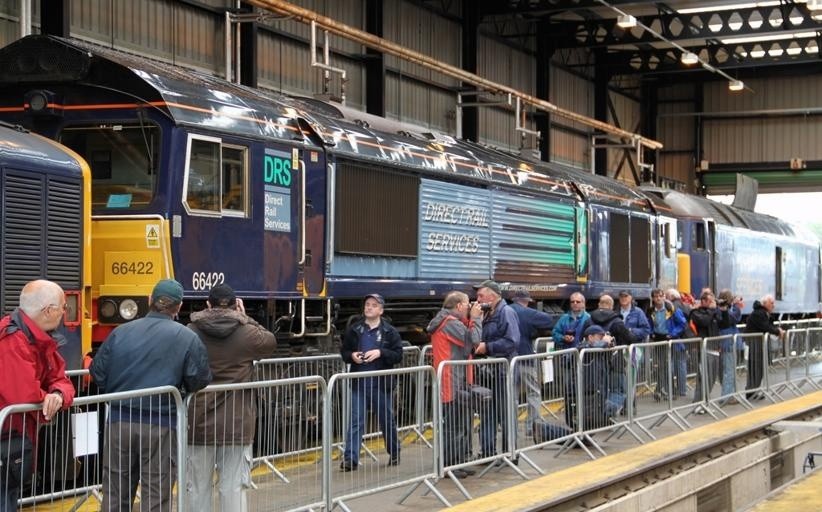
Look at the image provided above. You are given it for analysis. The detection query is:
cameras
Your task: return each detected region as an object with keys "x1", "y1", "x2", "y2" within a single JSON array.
[
  {"x1": 470, "y1": 300, "x2": 494, "y2": 311},
  {"x1": 714, "y1": 296, "x2": 729, "y2": 310}
]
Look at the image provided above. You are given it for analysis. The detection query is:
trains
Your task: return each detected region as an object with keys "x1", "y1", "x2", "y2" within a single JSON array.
[{"x1": 0, "y1": 32, "x2": 820, "y2": 503}]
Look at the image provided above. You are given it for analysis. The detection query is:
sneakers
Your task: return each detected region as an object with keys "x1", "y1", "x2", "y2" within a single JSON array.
[
  {"x1": 693, "y1": 406, "x2": 715, "y2": 414},
  {"x1": 533, "y1": 418, "x2": 543, "y2": 450},
  {"x1": 340, "y1": 459, "x2": 358, "y2": 471},
  {"x1": 654, "y1": 387, "x2": 687, "y2": 400},
  {"x1": 443, "y1": 452, "x2": 519, "y2": 479},
  {"x1": 719, "y1": 393, "x2": 765, "y2": 403},
  {"x1": 389, "y1": 450, "x2": 401, "y2": 466}
]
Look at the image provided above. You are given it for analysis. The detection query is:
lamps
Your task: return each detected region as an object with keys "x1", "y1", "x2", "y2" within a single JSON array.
[{"x1": 599, "y1": 0, "x2": 755, "y2": 93}]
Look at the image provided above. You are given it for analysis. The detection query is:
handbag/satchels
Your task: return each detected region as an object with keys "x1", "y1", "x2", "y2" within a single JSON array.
[
  {"x1": 0, "y1": 429, "x2": 35, "y2": 491},
  {"x1": 456, "y1": 383, "x2": 492, "y2": 410}
]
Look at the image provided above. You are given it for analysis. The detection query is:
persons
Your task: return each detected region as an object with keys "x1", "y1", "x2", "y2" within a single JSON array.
[
  {"x1": 90, "y1": 278, "x2": 212, "y2": 512},
  {"x1": 340, "y1": 293, "x2": 403, "y2": 470},
  {"x1": 0, "y1": 278, "x2": 76, "y2": 512},
  {"x1": 429, "y1": 279, "x2": 519, "y2": 481},
  {"x1": 182, "y1": 283, "x2": 277, "y2": 512},
  {"x1": 510, "y1": 287, "x2": 783, "y2": 449}
]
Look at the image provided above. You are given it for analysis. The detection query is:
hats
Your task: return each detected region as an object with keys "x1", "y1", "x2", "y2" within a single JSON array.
[
  {"x1": 208, "y1": 283, "x2": 236, "y2": 307},
  {"x1": 472, "y1": 280, "x2": 502, "y2": 295},
  {"x1": 152, "y1": 280, "x2": 184, "y2": 304},
  {"x1": 512, "y1": 287, "x2": 533, "y2": 302},
  {"x1": 619, "y1": 290, "x2": 632, "y2": 296},
  {"x1": 364, "y1": 293, "x2": 385, "y2": 304},
  {"x1": 584, "y1": 325, "x2": 608, "y2": 336}
]
